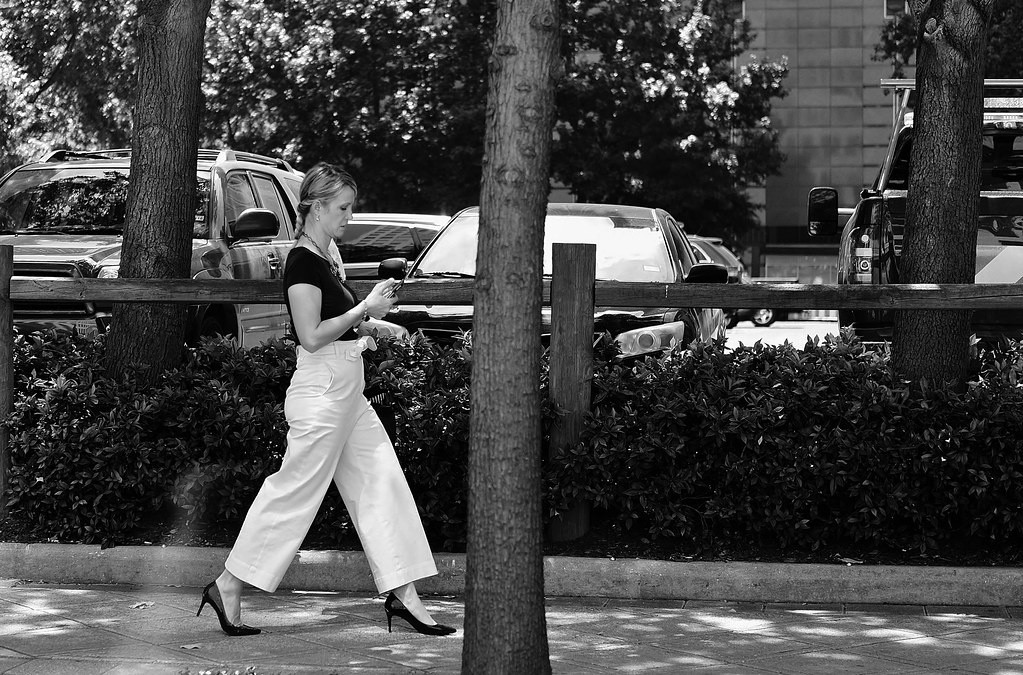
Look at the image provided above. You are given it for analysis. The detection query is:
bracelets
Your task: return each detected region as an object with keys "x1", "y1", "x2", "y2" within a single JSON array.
[{"x1": 360, "y1": 299, "x2": 367, "y2": 321}]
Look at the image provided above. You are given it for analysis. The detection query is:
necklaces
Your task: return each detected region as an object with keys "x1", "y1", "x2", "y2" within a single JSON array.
[{"x1": 301, "y1": 230, "x2": 339, "y2": 276}]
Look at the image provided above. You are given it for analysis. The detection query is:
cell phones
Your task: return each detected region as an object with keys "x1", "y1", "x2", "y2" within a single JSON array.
[{"x1": 385, "y1": 279, "x2": 404, "y2": 298}]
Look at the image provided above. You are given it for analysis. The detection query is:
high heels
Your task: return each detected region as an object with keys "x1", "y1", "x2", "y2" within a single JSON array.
[
  {"x1": 197, "y1": 580, "x2": 261, "y2": 636},
  {"x1": 384, "y1": 591, "x2": 456, "y2": 636}
]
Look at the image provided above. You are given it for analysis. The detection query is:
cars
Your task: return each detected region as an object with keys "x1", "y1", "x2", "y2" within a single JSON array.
[
  {"x1": 334, "y1": 214, "x2": 452, "y2": 305},
  {"x1": 377, "y1": 203, "x2": 729, "y2": 371}
]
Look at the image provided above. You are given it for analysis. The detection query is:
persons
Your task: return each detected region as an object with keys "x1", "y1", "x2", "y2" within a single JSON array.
[{"x1": 195, "y1": 161, "x2": 459, "y2": 637}]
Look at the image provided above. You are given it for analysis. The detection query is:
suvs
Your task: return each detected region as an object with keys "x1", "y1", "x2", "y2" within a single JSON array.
[
  {"x1": 686, "y1": 236, "x2": 745, "y2": 329},
  {"x1": 809, "y1": 76, "x2": 1023, "y2": 344},
  {"x1": 0, "y1": 147, "x2": 306, "y2": 357}
]
